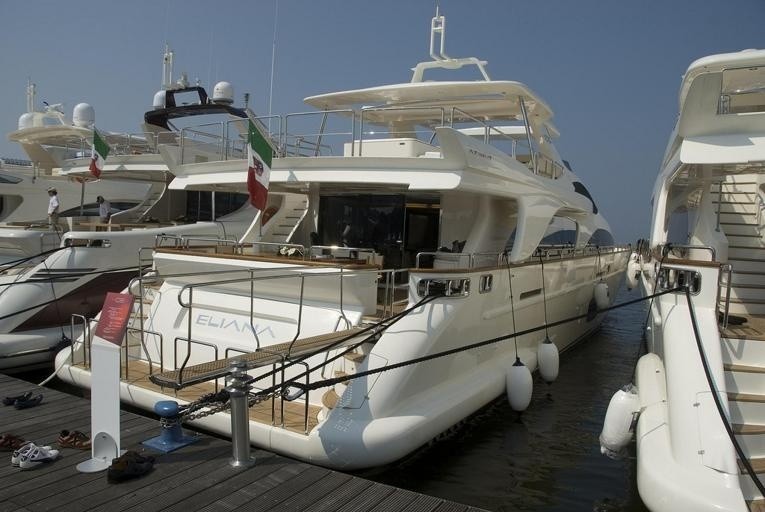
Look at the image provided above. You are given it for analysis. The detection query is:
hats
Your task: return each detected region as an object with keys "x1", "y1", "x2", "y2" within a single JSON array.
[
  {"x1": 96, "y1": 196, "x2": 102, "y2": 203},
  {"x1": 45, "y1": 186, "x2": 56, "y2": 191}
]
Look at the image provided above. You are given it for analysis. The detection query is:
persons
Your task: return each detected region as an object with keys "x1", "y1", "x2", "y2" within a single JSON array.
[
  {"x1": 96, "y1": 196, "x2": 112, "y2": 231},
  {"x1": 47, "y1": 186, "x2": 59, "y2": 231}
]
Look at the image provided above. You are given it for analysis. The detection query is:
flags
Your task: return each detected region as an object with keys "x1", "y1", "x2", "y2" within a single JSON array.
[
  {"x1": 247, "y1": 119, "x2": 272, "y2": 211},
  {"x1": 89, "y1": 130, "x2": 109, "y2": 178}
]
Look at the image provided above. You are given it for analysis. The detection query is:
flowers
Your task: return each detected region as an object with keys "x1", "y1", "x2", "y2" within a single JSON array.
[{"x1": 278, "y1": 247, "x2": 301, "y2": 257}]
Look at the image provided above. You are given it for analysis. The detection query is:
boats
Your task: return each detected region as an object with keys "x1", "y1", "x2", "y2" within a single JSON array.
[{"x1": 599, "y1": 48, "x2": 765, "y2": 512}]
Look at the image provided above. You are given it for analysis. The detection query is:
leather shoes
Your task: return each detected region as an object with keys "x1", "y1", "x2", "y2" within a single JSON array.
[{"x1": 107, "y1": 450, "x2": 154, "y2": 483}]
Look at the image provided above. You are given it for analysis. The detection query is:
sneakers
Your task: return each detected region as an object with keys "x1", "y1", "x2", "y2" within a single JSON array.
[
  {"x1": 3, "y1": 392, "x2": 42, "y2": 410},
  {"x1": 0, "y1": 430, "x2": 92, "y2": 469}
]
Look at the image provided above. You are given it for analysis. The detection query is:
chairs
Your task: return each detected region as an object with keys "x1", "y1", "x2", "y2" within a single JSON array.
[{"x1": 310, "y1": 246, "x2": 385, "y2": 285}]
